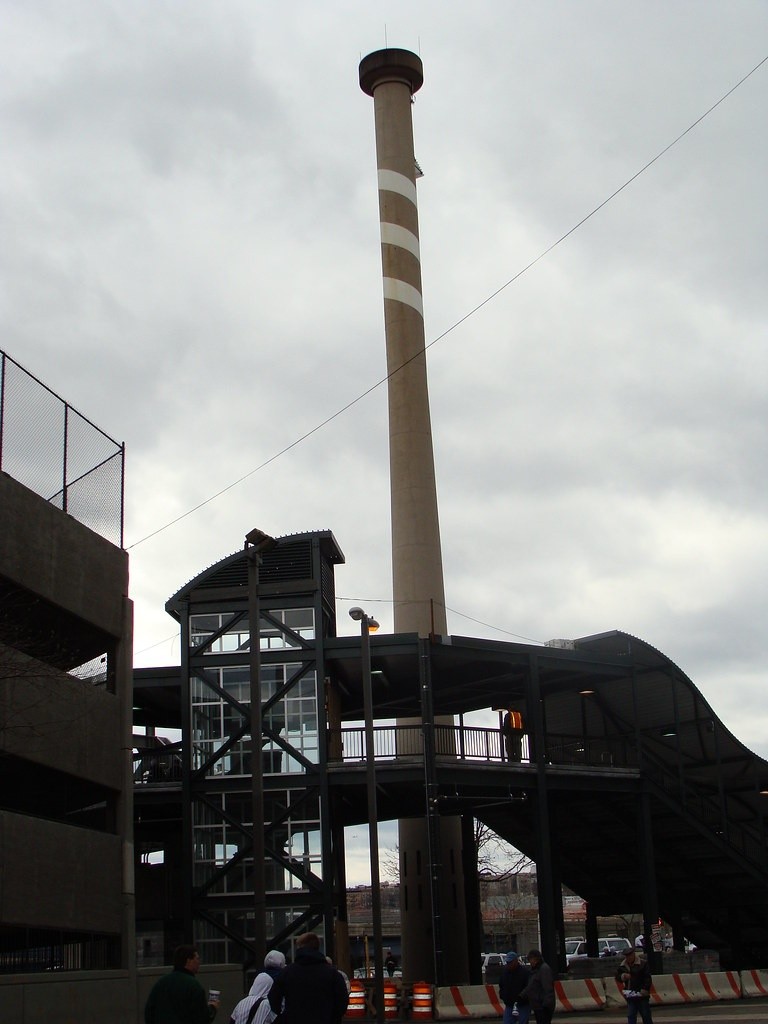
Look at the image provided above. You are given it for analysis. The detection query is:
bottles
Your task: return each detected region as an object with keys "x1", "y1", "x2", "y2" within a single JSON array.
[{"x1": 512, "y1": 1002, "x2": 519, "y2": 1016}]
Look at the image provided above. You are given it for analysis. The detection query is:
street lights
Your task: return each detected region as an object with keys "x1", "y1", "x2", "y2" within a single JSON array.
[
  {"x1": 349, "y1": 607, "x2": 386, "y2": 1024},
  {"x1": 244, "y1": 528, "x2": 279, "y2": 974}
]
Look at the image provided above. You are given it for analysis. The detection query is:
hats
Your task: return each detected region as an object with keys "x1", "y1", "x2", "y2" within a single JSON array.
[
  {"x1": 296, "y1": 932, "x2": 320, "y2": 950},
  {"x1": 264, "y1": 950, "x2": 283, "y2": 969},
  {"x1": 326, "y1": 957, "x2": 333, "y2": 965},
  {"x1": 388, "y1": 952, "x2": 392, "y2": 955}
]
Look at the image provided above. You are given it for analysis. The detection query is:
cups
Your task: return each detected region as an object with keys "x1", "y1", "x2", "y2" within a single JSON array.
[{"x1": 208, "y1": 990, "x2": 220, "y2": 1004}]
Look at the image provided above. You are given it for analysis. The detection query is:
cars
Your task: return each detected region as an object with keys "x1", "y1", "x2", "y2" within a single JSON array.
[
  {"x1": 390, "y1": 907, "x2": 400, "y2": 913},
  {"x1": 481, "y1": 952, "x2": 522, "y2": 973},
  {"x1": 564, "y1": 936, "x2": 633, "y2": 966},
  {"x1": 664, "y1": 932, "x2": 698, "y2": 953},
  {"x1": 517, "y1": 956, "x2": 531, "y2": 965},
  {"x1": 353, "y1": 967, "x2": 402, "y2": 978}
]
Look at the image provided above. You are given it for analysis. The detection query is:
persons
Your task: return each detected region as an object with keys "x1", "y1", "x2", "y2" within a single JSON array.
[
  {"x1": 504, "y1": 703, "x2": 525, "y2": 763},
  {"x1": 385, "y1": 952, "x2": 396, "y2": 977},
  {"x1": 147, "y1": 757, "x2": 182, "y2": 783},
  {"x1": 229, "y1": 932, "x2": 351, "y2": 1024},
  {"x1": 615, "y1": 948, "x2": 653, "y2": 1024},
  {"x1": 499, "y1": 948, "x2": 556, "y2": 1024},
  {"x1": 601, "y1": 946, "x2": 617, "y2": 959},
  {"x1": 163, "y1": 943, "x2": 181, "y2": 966},
  {"x1": 144, "y1": 945, "x2": 221, "y2": 1024},
  {"x1": 653, "y1": 940, "x2": 673, "y2": 953}
]
